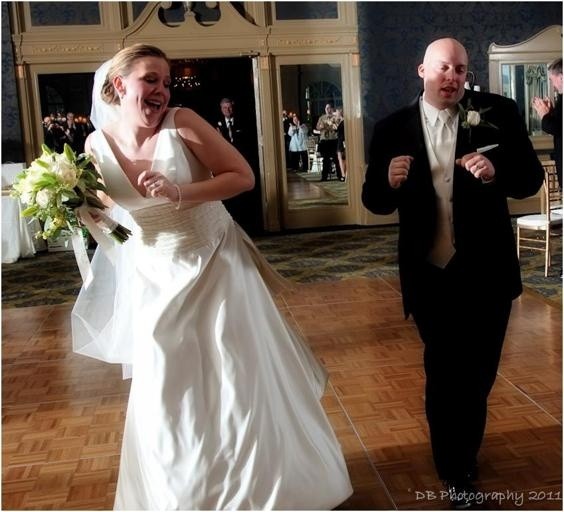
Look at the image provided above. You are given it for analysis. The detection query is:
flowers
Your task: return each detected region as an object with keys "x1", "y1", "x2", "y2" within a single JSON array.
[{"x1": 9, "y1": 144, "x2": 133, "y2": 245}]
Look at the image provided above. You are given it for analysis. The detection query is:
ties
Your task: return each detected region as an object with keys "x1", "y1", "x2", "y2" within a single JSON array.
[
  {"x1": 227, "y1": 120, "x2": 234, "y2": 143},
  {"x1": 436, "y1": 109, "x2": 451, "y2": 165}
]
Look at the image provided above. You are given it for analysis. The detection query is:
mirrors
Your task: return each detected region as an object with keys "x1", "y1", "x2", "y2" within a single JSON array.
[
  {"x1": 274, "y1": 53, "x2": 351, "y2": 212},
  {"x1": 499, "y1": 62, "x2": 555, "y2": 142}
]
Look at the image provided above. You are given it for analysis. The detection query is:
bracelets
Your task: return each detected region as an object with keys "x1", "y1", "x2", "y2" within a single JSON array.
[{"x1": 170, "y1": 184, "x2": 181, "y2": 211}]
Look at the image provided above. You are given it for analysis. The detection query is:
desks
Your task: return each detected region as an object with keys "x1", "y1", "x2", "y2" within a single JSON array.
[{"x1": 1, "y1": 185, "x2": 46, "y2": 265}]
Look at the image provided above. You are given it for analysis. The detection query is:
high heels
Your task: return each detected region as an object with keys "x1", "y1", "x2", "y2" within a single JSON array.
[{"x1": 341, "y1": 176, "x2": 345, "y2": 182}]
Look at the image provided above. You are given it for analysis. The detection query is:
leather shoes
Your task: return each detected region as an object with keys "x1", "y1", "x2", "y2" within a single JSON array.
[{"x1": 442, "y1": 479, "x2": 477, "y2": 511}]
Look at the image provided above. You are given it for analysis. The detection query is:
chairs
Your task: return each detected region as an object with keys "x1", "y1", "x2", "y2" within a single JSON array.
[{"x1": 516, "y1": 160, "x2": 563, "y2": 278}]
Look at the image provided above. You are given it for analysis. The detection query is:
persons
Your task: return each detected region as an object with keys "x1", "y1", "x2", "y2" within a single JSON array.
[
  {"x1": 213, "y1": 96, "x2": 259, "y2": 230},
  {"x1": 360, "y1": 38, "x2": 547, "y2": 509},
  {"x1": 40, "y1": 110, "x2": 94, "y2": 159},
  {"x1": 532, "y1": 57, "x2": 562, "y2": 279},
  {"x1": 282, "y1": 105, "x2": 346, "y2": 182},
  {"x1": 73, "y1": 43, "x2": 355, "y2": 511}
]
